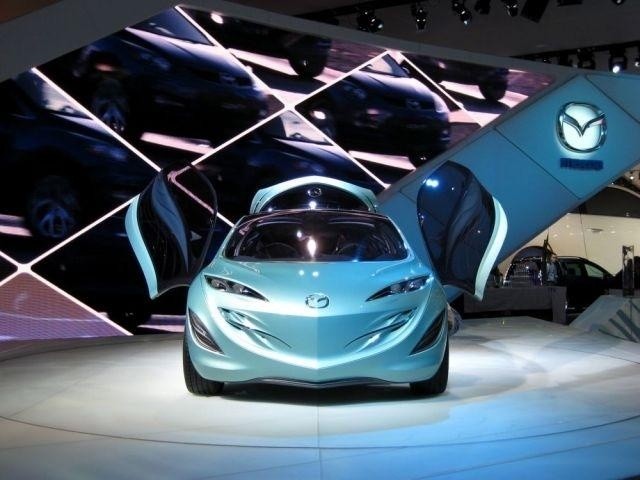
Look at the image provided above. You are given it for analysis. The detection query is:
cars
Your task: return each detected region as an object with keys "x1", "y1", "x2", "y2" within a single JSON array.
[
  {"x1": 505, "y1": 256, "x2": 628, "y2": 319},
  {"x1": 122, "y1": 159, "x2": 510, "y2": 397}
]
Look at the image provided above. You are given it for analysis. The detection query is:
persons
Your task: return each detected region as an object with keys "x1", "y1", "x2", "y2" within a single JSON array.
[{"x1": 538, "y1": 252, "x2": 559, "y2": 286}]
